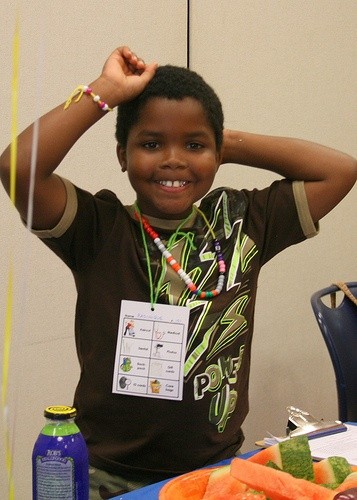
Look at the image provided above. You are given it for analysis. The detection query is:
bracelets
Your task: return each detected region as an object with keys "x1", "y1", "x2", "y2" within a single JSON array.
[{"x1": 65, "y1": 84, "x2": 112, "y2": 116}]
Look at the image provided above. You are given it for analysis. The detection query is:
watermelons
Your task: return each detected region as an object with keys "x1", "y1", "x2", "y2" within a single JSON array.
[
  {"x1": 312, "y1": 457, "x2": 350, "y2": 488},
  {"x1": 246, "y1": 435, "x2": 314, "y2": 481},
  {"x1": 228, "y1": 456, "x2": 337, "y2": 500}
]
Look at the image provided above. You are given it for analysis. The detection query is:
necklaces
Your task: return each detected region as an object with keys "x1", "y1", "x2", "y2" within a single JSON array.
[{"x1": 131, "y1": 198, "x2": 227, "y2": 298}]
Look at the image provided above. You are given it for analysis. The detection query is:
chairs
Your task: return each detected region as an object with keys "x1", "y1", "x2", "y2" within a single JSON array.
[{"x1": 310, "y1": 281, "x2": 357, "y2": 428}]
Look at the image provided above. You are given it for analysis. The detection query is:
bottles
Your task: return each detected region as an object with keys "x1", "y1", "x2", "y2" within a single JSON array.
[{"x1": 32, "y1": 406, "x2": 89, "y2": 500}]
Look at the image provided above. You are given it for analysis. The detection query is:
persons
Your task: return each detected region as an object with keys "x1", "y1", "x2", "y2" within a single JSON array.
[{"x1": 1, "y1": 44, "x2": 357, "y2": 500}]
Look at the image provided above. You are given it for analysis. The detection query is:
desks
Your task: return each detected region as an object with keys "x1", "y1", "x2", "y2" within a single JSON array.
[{"x1": 101, "y1": 422, "x2": 357, "y2": 500}]
